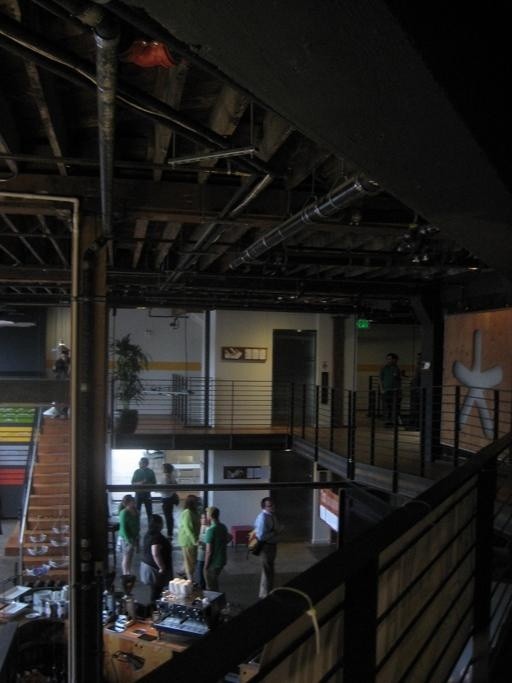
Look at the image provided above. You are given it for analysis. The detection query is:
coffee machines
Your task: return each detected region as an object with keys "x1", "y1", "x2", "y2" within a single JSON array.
[
  {"x1": 101, "y1": 572, "x2": 137, "y2": 628},
  {"x1": 152, "y1": 589, "x2": 225, "y2": 642}
]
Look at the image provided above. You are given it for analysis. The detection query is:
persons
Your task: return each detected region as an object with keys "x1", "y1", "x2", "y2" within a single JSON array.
[
  {"x1": 177, "y1": 493, "x2": 200, "y2": 580},
  {"x1": 159, "y1": 462, "x2": 180, "y2": 540},
  {"x1": 193, "y1": 512, "x2": 212, "y2": 589},
  {"x1": 247, "y1": 496, "x2": 287, "y2": 597},
  {"x1": 130, "y1": 456, "x2": 157, "y2": 519},
  {"x1": 202, "y1": 505, "x2": 233, "y2": 591},
  {"x1": 139, "y1": 513, "x2": 173, "y2": 611},
  {"x1": 118, "y1": 494, "x2": 143, "y2": 576},
  {"x1": 380, "y1": 352, "x2": 403, "y2": 427},
  {"x1": 50, "y1": 342, "x2": 71, "y2": 420}
]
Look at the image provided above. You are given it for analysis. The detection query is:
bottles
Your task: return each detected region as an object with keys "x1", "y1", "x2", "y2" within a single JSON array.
[{"x1": 39, "y1": 599, "x2": 67, "y2": 619}]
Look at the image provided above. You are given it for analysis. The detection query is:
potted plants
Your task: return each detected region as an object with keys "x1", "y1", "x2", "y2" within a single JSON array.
[{"x1": 108, "y1": 332, "x2": 153, "y2": 433}]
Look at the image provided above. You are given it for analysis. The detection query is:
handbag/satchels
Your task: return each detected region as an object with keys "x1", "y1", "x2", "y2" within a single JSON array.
[{"x1": 247, "y1": 525, "x2": 265, "y2": 556}]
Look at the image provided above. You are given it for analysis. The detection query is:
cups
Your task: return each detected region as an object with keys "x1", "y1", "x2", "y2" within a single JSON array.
[{"x1": 168, "y1": 577, "x2": 194, "y2": 596}]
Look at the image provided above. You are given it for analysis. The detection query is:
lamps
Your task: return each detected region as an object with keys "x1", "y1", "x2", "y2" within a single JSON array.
[{"x1": 111, "y1": 648, "x2": 145, "y2": 682}]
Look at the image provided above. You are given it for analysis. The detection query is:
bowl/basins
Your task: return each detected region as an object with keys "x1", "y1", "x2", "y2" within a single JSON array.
[{"x1": 25, "y1": 524, "x2": 68, "y2": 576}]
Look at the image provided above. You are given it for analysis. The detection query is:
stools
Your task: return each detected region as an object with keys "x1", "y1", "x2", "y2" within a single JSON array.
[
  {"x1": 231, "y1": 524, "x2": 254, "y2": 548},
  {"x1": 108, "y1": 521, "x2": 120, "y2": 552}
]
